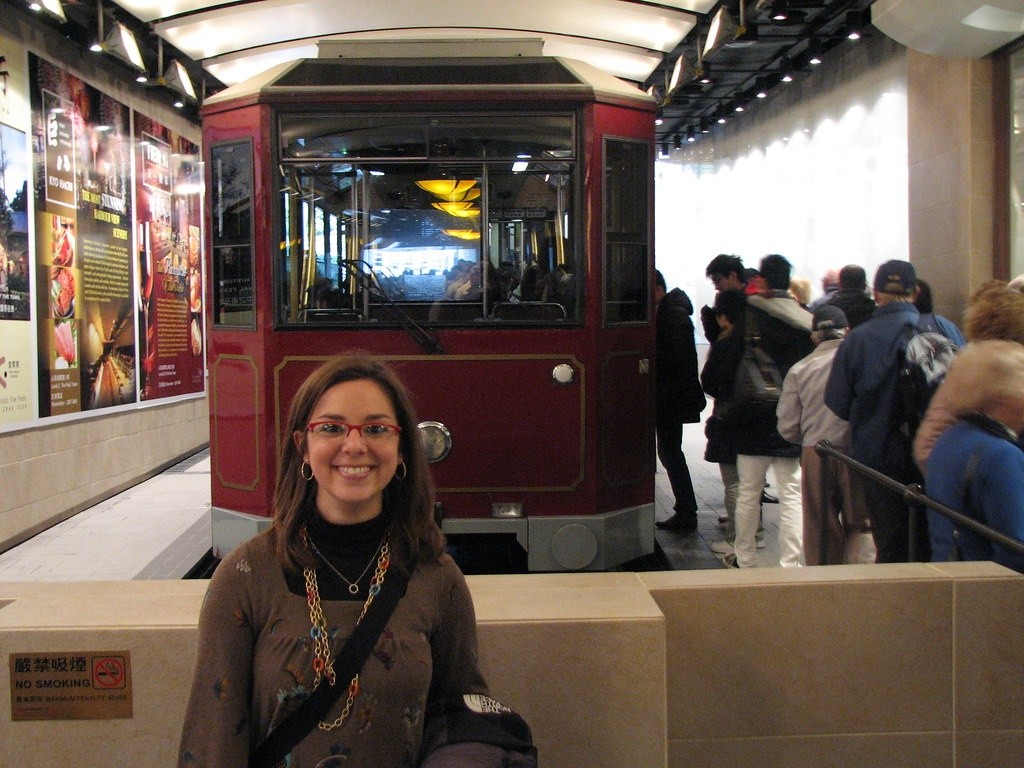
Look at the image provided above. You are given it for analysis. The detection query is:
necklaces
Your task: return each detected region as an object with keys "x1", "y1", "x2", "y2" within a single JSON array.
[{"x1": 300, "y1": 523, "x2": 391, "y2": 730}]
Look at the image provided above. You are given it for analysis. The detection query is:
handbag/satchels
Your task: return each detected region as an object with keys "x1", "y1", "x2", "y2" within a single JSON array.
[{"x1": 711, "y1": 312, "x2": 782, "y2": 426}]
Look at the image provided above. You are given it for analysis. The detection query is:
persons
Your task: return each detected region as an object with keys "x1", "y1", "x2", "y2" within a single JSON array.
[
  {"x1": 810, "y1": 264, "x2": 878, "y2": 329},
  {"x1": 914, "y1": 278, "x2": 966, "y2": 347},
  {"x1": 179, "y1": 356, "x2": 491, "y2": 768},
  {"x1": 700, "y1": 253, "x2": 812, "y2": 569},
  {"x1": 827, "y1": 259, "x2": 966, "y2": 563},
  {"x1": 656, "y1": 270, "x2": 706, "y2": 534},
  {"x1": 912, "y1": 287, "x2": 1024, "y2": 473},
  {"x1": 441, "y1": 259, "x2": 572, "y2": 311},
  {"x1": 777, "y1": 304, "x2": 869, "y2": 566},
  {"x1": 924, "y1": 339, "x2": 1024, "y2": 572}
]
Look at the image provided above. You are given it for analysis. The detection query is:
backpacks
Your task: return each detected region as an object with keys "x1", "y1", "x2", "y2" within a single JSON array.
[{"x1": 898, "y1": 312, "x2": 960, "y2": 433}]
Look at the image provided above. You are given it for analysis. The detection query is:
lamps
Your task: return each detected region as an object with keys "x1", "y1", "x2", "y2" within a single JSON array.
[
  {"x1": 88, "y1": 34, "x2": 102, "y2": 55},
  {"x1": 442, "y1": 227, "x2": 472, "y2": 237},
  {"x1": 157, "y1": 37, "x2": 200, "y2": 102},
  {"x1": 458, "y1": 232, "x2": 480, "y2": 240},
  {"x1": 133, "y1": 60, "x2": 151, "y2": 83},
  {"x1": 434, "y1": 187, "x2": 482, "y2": 200},
  {"x1": 428, "y1": 201, "x2": 474, "y2": 211},
  {"x1": 24, "y1": 0, "x2": 67, "y2": 23},
  {"x1": 97, "y1": 0, "x2": 147, "y2": 72},
  {"x1": 448, "y1": 207, "x2": 481, "y2": 218},
  {"x1": 416, "y1": 177, "x2": 478, "y2": 194},
  {"x1": 170, "y1": 89, "x2": 186, "y2": 109},
  {"x1": 656, "y1": 0, "x2": 862, "y2": 156}
]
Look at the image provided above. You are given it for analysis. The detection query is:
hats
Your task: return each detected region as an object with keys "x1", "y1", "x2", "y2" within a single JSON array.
[
  {"x1": 874, "y1": 259, "x2": 916, "y2": 297},
  {"x1": 811, "y1": 303, "x2": 848, "y2": 332}
]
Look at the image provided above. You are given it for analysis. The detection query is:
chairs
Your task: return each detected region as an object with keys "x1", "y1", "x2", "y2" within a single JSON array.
[
  {"x1": 492, "y1": 299, "x2": 566, "y2": 319},
  {"x1": 296, "y1": 309, "x2": 364, "y2": 321}
]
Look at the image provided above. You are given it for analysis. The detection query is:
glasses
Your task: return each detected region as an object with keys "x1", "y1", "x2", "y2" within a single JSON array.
[{"x1": 304, "y1": 421, "x2": 402, "y2": 443}]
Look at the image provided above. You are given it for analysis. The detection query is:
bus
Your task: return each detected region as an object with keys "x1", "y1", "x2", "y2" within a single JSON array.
[{"x1": 196, "y1": 34, "x2": 661, "y2": 572}]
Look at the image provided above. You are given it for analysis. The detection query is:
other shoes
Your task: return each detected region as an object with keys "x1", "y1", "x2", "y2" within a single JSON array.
[
  {"x1": 710, "y1": 530, "x2": 766, "y2": 553},
  {"x1": 655, "y1": 513, "x2": 698, "y2": 532},
  {"x1": 718, "y1": 514, "x2": 730, "y2": 522},
  {"x1": 722, "y1": 554, "x2": 739, "y2": 569}
]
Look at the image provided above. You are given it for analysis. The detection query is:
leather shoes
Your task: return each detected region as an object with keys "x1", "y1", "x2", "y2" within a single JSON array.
[{"x1": 762, "y1": 490, "x2": 779, "y2": 503}]
